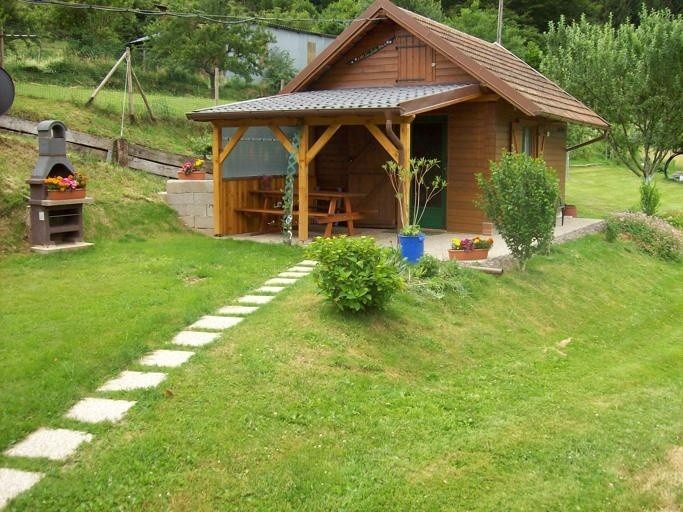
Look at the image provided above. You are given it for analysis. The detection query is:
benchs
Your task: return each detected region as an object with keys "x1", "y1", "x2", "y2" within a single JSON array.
[
  {"x1": 235, "y1": 208, "x2": 329, "y2": 219},
  {"x1": 295, "y1": 204, "x2": 379, "y2": 214}
]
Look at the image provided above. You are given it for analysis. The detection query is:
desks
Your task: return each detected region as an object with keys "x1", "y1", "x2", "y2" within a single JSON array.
[{"x1": 250, "y1": 188, "x2": 368, "y2": 240}]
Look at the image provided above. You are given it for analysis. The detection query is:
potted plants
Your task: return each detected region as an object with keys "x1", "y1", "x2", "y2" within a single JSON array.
[{"x1": 381, "y1": 156, "x2": 449, "y2": 266}]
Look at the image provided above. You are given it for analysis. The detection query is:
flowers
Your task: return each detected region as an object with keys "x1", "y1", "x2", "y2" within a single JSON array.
[
  {"x1": 450, "y1": 236, "x2": 494, "y2": 253},
  {"x1": 44, "y1": 171, "x2": 88, "y2": 192},
  {"x1": 182, "y1": 158, "x2": 205, "y2": 176}
]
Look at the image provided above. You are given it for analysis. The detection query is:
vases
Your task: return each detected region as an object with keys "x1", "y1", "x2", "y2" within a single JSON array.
[
  {"x1": 178, "y1": 172, "x2": 205, "y2": 180},
  {"x1": 447, "y1": 249, "x2": 489, "y2": 260},
  {"x1": 47, "y1": 188, "x2": 86, "y2": 200}
]
